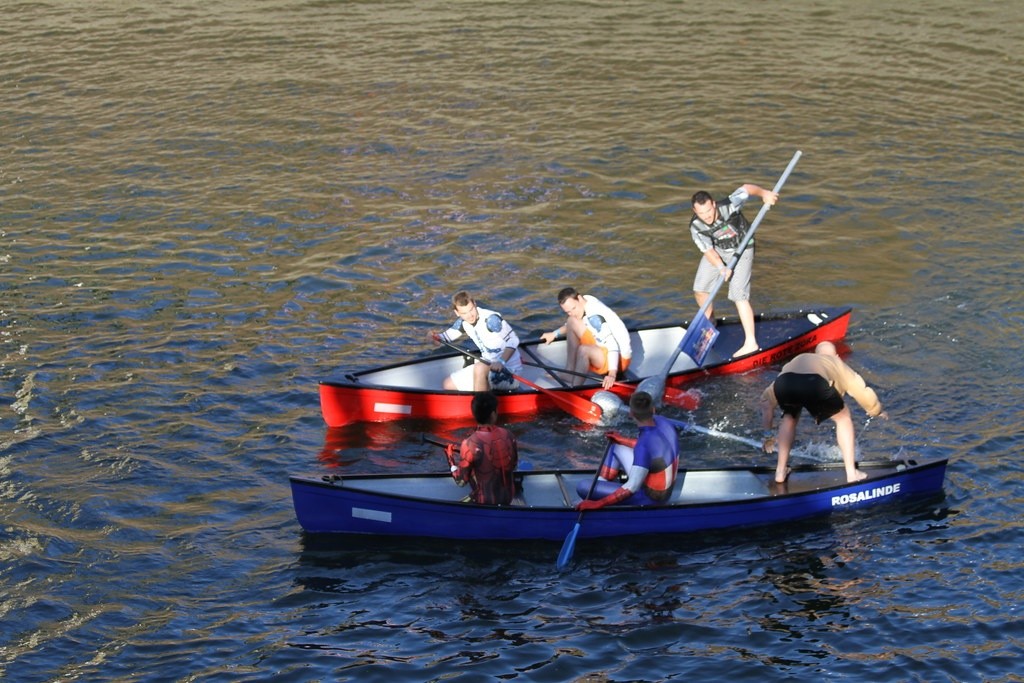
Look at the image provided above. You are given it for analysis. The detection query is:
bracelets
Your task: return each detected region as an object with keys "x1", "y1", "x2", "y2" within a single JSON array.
[
  {"x1": 764, "y1": 432, "x2": 771, "y2": 435},
  {"x1": 554, "y1": 329, "x2": 560, "y2": 338},
  {"x1": 498, "y1": 358, "x2": 505, "y2": 365}
]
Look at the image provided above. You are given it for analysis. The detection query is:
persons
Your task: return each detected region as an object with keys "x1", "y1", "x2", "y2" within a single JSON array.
[
  {"x1": 444, "y1": 393, "x2": 518, "y2": 505},
  {"x1": 760, "y1": 341, "x2": 889, "y2": 483},
  {"x1": 430, "y1": 291, "x2": 523, "y2": 391},
  {"x1": 540, "y1": 287, "x2": 633, "y2": 390},
  {"x1": 575, "y1": 391, "x2": 679, "y2": 510},
  {"x1": 689, "y1": 184, "x2": 779, "y2": 357}
]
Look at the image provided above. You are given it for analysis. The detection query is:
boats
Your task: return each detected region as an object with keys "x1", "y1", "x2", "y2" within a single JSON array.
[
  {"x1": 290, "y1": 456, "x2": 948, "y2": 540},
  {"x1": 318, "y1": 306, "x2": 855, "y2": 428}
]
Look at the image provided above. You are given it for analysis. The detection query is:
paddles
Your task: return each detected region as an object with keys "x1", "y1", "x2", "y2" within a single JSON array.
[
  {"x1": 419, "y1": 434, "x2": 534, "y2": 474},
  {"x1": 555, "y1": 431, "x2": 615, "y2": 572},
  {"x1": 431, "y1": 332, "x2": 604, "y2": 425},
  {"x1": 520, "y1": 353, "x2": 701, "y2": 412}
]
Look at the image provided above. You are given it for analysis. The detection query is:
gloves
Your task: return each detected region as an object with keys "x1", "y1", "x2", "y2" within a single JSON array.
[
  {"x1": 444, "y1": 443, "x2": 454, "y2": 459},
  {"x1": 576, "y1": 486, "x2": 632, "y2": 510},
  {"x1": 605, "y1": 431, "x2": 637, "y2": 447}
]
